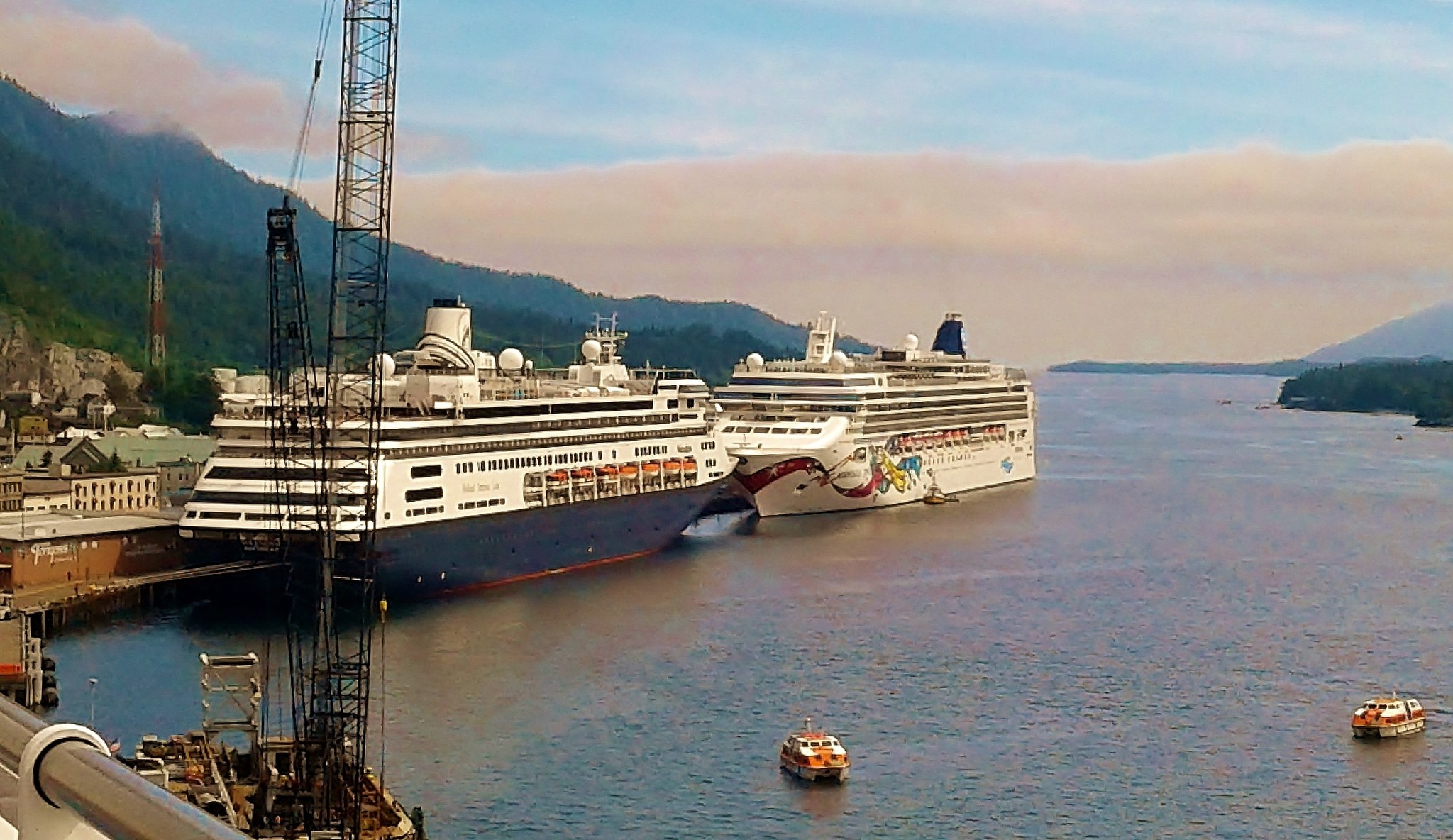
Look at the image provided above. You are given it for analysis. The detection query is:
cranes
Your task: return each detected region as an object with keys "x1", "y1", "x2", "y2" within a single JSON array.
[
  {"x1": 266, "y1": 0, "x2": 402, "y2": 840},
  {"x1": 147, "y1": 193, "x2": 166, "y2": 418}
]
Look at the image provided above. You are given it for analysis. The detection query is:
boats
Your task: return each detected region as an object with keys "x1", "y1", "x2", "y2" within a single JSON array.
[
  {"x1": 896, "y1": 426, "x2": 1004, "y2": 447},
  {"x1": 180, "y1": 295, "x2": 739, "y2": 600},
  {"x1": 525, "y1": 461, "x2": 698, "y2": 496},
  {"x1": 1350, "y1": 685, "x2": 1427, "y2": 737},
  {"x1": 710, "y1": 311, "x2": 1039, "y2": 518},
  {"x1": 924, "y1": 475, "x2": 959, "y2": 504},
  {"x1": 119, "y1": 653, "x2": 427, "y2": 840},
  {"x1": 780, "y1": 717, "x2": 852, "y2": 786}
]
